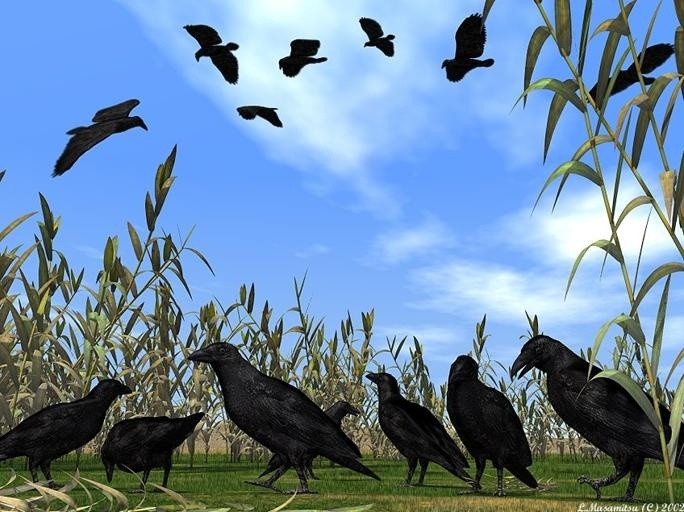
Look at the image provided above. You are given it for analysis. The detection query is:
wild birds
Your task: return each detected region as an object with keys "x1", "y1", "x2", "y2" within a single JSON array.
[
  {"x1": 586, "y1": 43, "x2": 676, "y2": 104},
  {"x1": 0, "y1": 335, "x2": 684, "y2": 502},
  {"x1": 50, "y1": 99, "x2": 148, "y2": 178},
  {"x1": 237, "y1": 106, "x2": 283, "y2": 128},
  {"x1": 184, "y1": 13, "x2": 494, "y2": 84}
]
